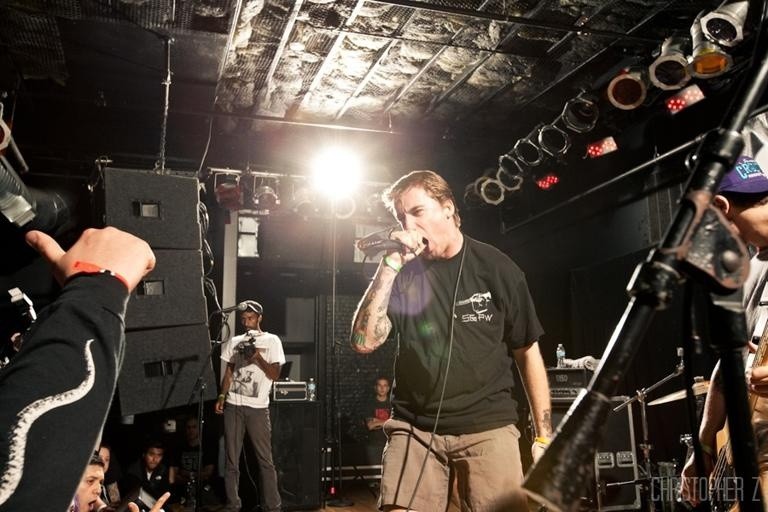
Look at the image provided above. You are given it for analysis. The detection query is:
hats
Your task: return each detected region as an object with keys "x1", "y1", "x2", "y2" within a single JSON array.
[
  {"x1": 716, "y1": 156, "x2": 768, "y2": 194},
  {"x1": 245, "y1": 301, "x2": 263, "y2": 315}
]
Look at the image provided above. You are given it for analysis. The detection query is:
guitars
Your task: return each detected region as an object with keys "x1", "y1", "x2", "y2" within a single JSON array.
[{"x1": 707, "y1": 319, "x2": 768, "y2": 512}]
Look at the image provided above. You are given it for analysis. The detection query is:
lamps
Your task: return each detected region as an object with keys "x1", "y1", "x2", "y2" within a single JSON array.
[
  {"x1": 685, "y1": 11, "x2": 733, "y2": 79},
  {"x1": 515, "y1": 123, "x2": 547, "y2": 167},
  {"x1": 647, "y1": 35, "x2": 692, "y2": 90},
  {"x1": 463, "y1": 147, "x2": 526, "y2": 208},
  {"x1": 539, "y1": 115, "x2": 573, "y2": 156},
  {"x1": 701, "y1": 1, "x2": 747, "y2": 45},
  {"x1": 608, "y1": 65, "x2": 647, "y2": 110},
  {"x1": 561, "y1": 93, "x2": 601, "y2": 134},
  {"x1": 211, "y1": 167, "x2": 385, "y2": 225}
]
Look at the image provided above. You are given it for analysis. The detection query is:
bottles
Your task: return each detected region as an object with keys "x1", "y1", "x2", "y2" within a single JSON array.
[
  {"x1": 308, "y1": 378, "x2": 317, "y2": 401},
  {"x1": 556, "y1": 344, "x2": 567, "y2": 369}
]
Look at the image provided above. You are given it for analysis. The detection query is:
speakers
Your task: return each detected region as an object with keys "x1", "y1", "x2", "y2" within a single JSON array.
[
  {"x1": 268, "y1": 401, "x2": 323, "y2": 512},
  {"x1": 95, "y1": 168, "x2": 218, "y2": 420}
]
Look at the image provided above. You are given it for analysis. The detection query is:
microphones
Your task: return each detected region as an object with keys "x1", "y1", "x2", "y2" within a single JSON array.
[{"x1": 373, "y1": 240, "x2": 405, "y2": 252}]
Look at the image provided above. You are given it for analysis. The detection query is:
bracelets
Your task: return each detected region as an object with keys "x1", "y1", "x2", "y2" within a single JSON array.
[
  {"x1": 533, "y1": 437, "x2": 552, "y2": 445},
  {"x1": 217, "y1": 393, "x2": 225, "y2": 399},
  {"x1": 71, "y1": 259, "x2": 131, "y2": 294},
  {"x1": 699, "y1": 442, "x2": 714, "y2": 456},
  {"x1": 381, "y1": 255, "x2": 402, "y2": 274}
]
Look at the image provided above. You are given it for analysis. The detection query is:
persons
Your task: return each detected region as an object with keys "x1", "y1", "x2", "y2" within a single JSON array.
[
  {"x1": 213, "y1": 299, "x2": 288, "y2": 512},
  {"x1": 0, "y1": 224, "x2": 157, "y2": 511},
  {"x1": 680, "y1": 155, "x2": 768, "y2": 511},
  {"x1": 169, "y1": 417, "x2": 208, "y2": 472},
  {"x1": 123, "y1": 438, "x2": 179, "y2": 512},
  {"x1": 364, "y1": 376, "x2": 393, "y2": 431},
  {"x1": 67, "y1": 450, "x2": 173, "y2": 512},
  {"x1": 97, "y1": 442, "x2": 122, "y2": 506},
  {"x1": 344, "y1": 169, "x2": 554, "y2": 510}
]
